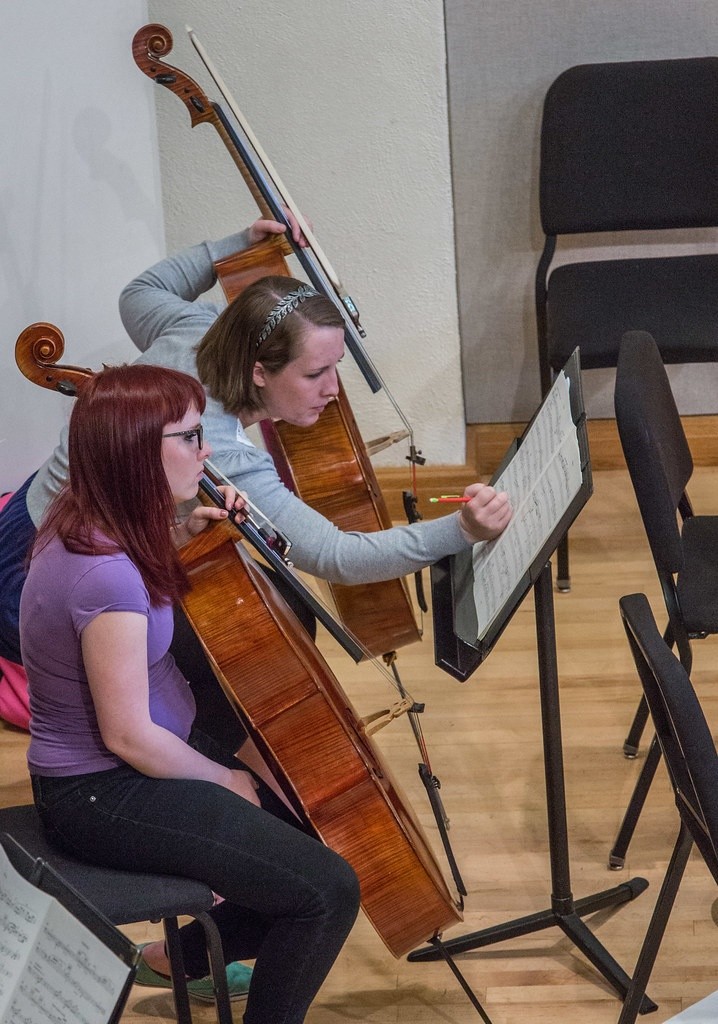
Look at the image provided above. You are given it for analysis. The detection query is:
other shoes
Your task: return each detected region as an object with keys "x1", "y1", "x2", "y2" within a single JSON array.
[{"x1": 133, "y1": 940, "x2": 254, "y2": 1005}]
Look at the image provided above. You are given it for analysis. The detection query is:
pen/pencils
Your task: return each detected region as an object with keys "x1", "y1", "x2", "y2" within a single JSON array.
[{"x1": 429, "y1": 494, "x2": 474, "y2": 503}]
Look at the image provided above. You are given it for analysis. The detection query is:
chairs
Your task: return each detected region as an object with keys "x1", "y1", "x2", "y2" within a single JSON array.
[
  {"x1": 616, "y1": 593, "x2": 718, "y2": 1024},
  {"x1": 0, "y1": 804, "x2": 233, "y2": 1024},
  {"x1": 606, "y1": 330, "x2": 718, "y2": 870},
  {"x1": 533, "y1": 57, "x2": 718, "y2": 593}
]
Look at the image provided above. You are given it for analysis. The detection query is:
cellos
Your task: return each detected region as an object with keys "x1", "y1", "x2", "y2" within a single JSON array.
[
  {"x1": 127, "y1": 23, "x2": 477, "y2": 902},
  {"x1": 12, "y1": 319, "x2": 499, "y2": 1024}
]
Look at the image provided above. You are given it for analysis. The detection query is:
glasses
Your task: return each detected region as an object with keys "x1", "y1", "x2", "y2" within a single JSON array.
[{"x1": 161, "y1": 424, "x2": 204, "y2": 451}]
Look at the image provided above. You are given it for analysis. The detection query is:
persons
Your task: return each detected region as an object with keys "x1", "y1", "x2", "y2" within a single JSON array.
[
  {"x1": 18, "y1": 362, "x2": 360, "y2": 1023},
  {"x1": 1, "y1": 205, "x2": 515, "y2": 823}
]
